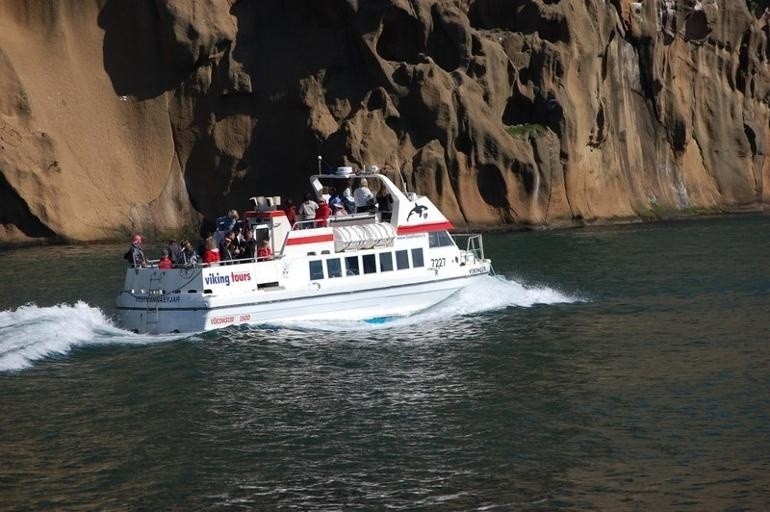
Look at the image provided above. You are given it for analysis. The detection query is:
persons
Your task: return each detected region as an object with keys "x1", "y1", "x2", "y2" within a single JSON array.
[
  {"x1": 276, "y1": 179, "x2": 394, "y2": 230},
  {"x1": 158, "y1": 210, "x2": 273, "y2": 293},
  {"x1": 123, "y1": 234, "x2": 145, "y2": 268}
]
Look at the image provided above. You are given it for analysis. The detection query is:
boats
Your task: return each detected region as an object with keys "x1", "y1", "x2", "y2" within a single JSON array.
[{"x1": 115, "y1": 147, "x2": 496, "y2": 334}]
